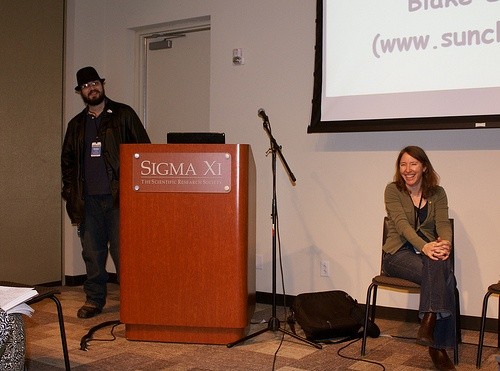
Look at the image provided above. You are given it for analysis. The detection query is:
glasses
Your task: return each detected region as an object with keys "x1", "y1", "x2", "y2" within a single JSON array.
[{"x1": 81, "y1": 81, "x2": 101, "y2": 90}]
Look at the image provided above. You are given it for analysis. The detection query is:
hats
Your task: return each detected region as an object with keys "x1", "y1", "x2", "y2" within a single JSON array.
[{"x1": 74, "y1": 67, "x2": 106, "y2": 91}]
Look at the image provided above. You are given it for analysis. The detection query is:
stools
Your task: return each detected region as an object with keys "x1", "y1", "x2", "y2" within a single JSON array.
[{"x1": 477, "y1": 283, "x2": 500, "y2": 368}]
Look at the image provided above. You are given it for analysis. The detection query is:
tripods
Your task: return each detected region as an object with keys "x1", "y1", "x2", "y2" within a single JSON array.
[{"x1": 226, "y1": 121, "x2": 323, "y2": 349}]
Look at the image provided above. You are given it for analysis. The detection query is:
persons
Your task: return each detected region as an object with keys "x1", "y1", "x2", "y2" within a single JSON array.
[
  {"x1": 60, "y1": 66, "x2": 151, "y2": 319},
  {"x1": 381, "y1": 145, "x2": 459, "y2": 371},
  {"x1": 0, "y1": 307, "x2": 24, "y2": 371}
]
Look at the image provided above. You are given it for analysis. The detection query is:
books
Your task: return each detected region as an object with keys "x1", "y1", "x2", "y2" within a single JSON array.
[{"x1": 0, "y1": 284, "x2": 40, "y2": 318}]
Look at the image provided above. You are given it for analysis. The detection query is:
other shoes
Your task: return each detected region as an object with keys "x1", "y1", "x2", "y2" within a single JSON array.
[
  {"x1": 428, "y1": 347, "x2": 456, "y2": 371},
  {"x1": 416, "y1": 313, "x2": 438, "y2": 346},
  {"x1": 77, "y1": 302, "x2": 105, "y2": 318}
]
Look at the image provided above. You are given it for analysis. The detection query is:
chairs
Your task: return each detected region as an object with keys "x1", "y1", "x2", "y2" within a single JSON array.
[
  {"x1": 360, "y1": 217, "x2": 462, "y2": 366},
  {"x1": 0, "y1": 279, "x2": 72, "y2": 371}
]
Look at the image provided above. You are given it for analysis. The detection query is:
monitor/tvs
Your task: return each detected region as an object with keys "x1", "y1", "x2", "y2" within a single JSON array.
[{"x1": 167, "y1": 133, "x2": 226, "y2": 145}]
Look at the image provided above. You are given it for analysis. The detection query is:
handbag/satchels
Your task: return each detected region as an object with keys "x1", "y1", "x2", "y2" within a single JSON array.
[{"x1": 288, "y1": 290, "x2": 380, "y2": 345}]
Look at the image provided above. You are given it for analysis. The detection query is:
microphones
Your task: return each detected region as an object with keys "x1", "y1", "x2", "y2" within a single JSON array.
[{"x1": 258, "y1": 108, "x2": 268, "y2": 121}]
